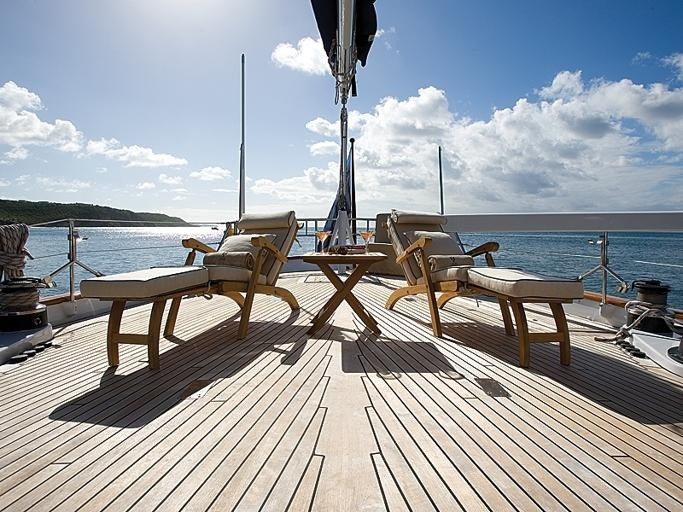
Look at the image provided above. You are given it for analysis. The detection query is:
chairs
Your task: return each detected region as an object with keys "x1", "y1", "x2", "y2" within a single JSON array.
[
  {"x1": 367, "y1": 207, "x2": 583, "y2": 370},
  {"x1": 79, "y1": 211, "x2": 298, "y2": 370}
]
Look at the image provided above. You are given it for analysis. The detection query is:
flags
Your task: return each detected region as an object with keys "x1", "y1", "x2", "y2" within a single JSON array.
[{"x1": 316, "y1": 145, "x2": 352, "y2": 251}]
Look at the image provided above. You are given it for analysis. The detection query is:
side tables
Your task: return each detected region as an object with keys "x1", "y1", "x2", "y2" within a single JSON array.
[{"x1": 303, "y1": 252, "x2": 388, "y2": 336}]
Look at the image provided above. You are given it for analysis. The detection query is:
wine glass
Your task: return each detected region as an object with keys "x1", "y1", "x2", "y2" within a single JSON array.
[
  {"x1": 314, "y1": 231, "x2": 332, "y2": 255},
  {"x1": 359, "y1": 230, "x2": 376, "y2": 255}
]
